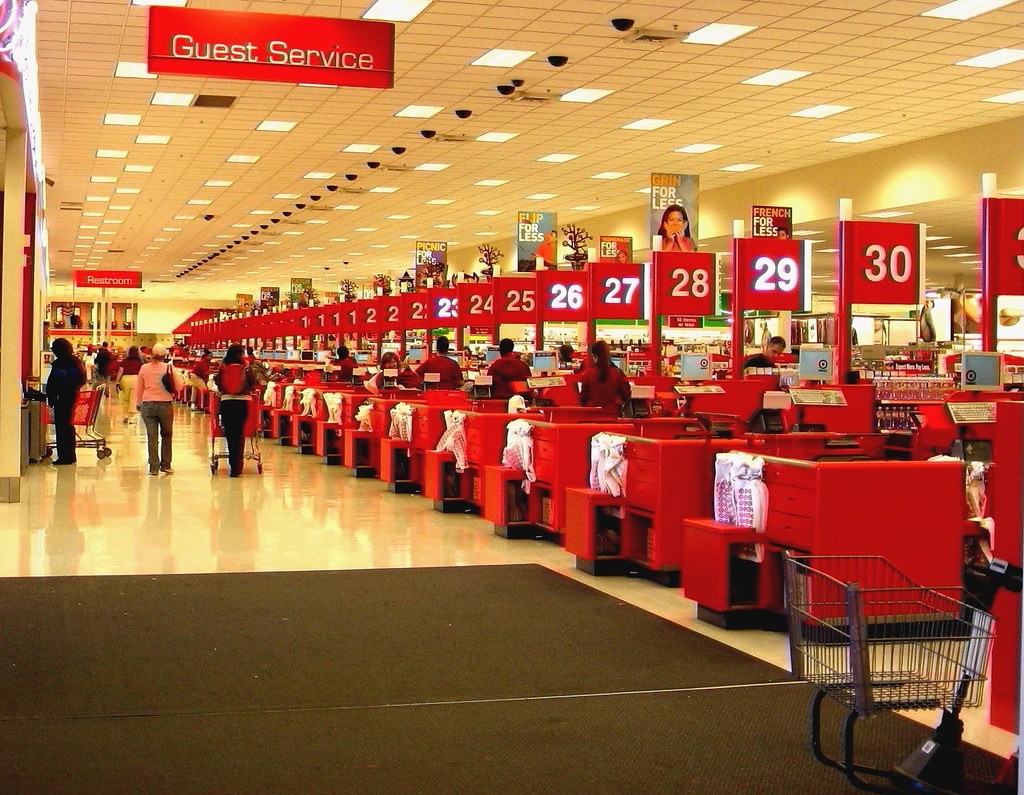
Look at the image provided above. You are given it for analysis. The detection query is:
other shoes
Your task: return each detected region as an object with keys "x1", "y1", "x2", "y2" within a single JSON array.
[
  {"x1": 230, "y1": 467, "x2": 242, "y2": 476},
  {"x1": 123, "y1": 415, "x2": 135, "y2": 424},
  {"x1": 149, "y1": 471, "x2": 158, "y2": 474},
  {"x1": 161, "y1": 467, "x2": 174, "y2": 473},
  {"x1": 52, "y1": 456, "x2": 76, "y2": 465}
]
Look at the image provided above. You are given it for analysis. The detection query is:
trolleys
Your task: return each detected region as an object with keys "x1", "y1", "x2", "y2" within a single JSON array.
[
  {"x1": 207, "y1": 387, "x2": 264, "y2": 475},
  {"x1": 41, "y1": 387, "x2": 112, "y2": 460}
]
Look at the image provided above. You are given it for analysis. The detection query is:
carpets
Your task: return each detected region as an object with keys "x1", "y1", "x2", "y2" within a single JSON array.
[{"x1": 0, "y1": 563, "x2": 1020, "y2": 795}]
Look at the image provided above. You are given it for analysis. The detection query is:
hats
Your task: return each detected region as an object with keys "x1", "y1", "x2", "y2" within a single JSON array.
[{"x1": 152, "y1": 343, "x2": 166, "y2": 356}]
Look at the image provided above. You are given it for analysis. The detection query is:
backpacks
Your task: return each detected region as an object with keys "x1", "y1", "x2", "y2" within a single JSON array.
[{"x1": 221, "y1": 363, "x2": 248, "y2": 394}]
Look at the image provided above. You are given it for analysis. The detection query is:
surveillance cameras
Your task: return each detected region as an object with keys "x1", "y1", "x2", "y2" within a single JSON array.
[
  {"x1": 325, "y1": 268, "x2": 329, "y2": 270},
  {"x1": 343, "y1": 262, "x2": 349, "y2": 264},
  {"x1": 176, "y1": 195, "x2": 322, "y2": 278},
  {"x1": 455, "y1": 110, "x2": 472, "y2": 119},
  {"x1": 366, "y1": 162, "x2": 380, "y2": 169},
  {"x1": 392, "y1": 147, "x2": 406, "y2": 154},
  {"x1": 497, "y1": 85, "x2": 515, "y2": 95},
  {"x1": 141, "y1": 290, "x2": 145, "y2": 291},
  {"x1": 420, "y1": 131, "x2": 436, "y2": 138},
  {"x1": 611, "y1": 19, "x2": 634, "y2": 31},
  {"x1": 346, "y1": 174, "x2": 357, "y2": 180},
  {"x1": 511, "y1": 79, "x2": 524, "y2": 87},
  {"x1": 547, "y1": 56, "x2": 569, "y2": 68},
  {"x1": 327, "y1": 186, "x2": 338, "y2": 191}
]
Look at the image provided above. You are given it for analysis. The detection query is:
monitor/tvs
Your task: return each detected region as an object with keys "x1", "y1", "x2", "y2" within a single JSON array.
[
  {"x1": 799, "y1": 347, "x2": 833, "y2": 381},
  {"x1": 196, "y1": 344, "x2": 625, "y2": 373},
  {"x1": 961, "y1": 353, "x2": 1004, "y2": 391},
  {"x1": 681, "y1": 353, "x2": 713, "y2": 381}
]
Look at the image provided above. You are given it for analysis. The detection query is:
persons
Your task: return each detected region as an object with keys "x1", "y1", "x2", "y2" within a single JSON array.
[
  {"x1": 531, "y1": 231, "x2": 557, "y2": 265},
  {"x1": 83, "y1": 344, "x2": 98, "y2": 385},
  {"x1": 615, "y1": 250, "x2": 630, "y2": 263},
  {"x1": 577, "y1": 340, "x2": 631, "y2": 418},
  {"x1": 94, "y1": 341, "x2": 113, "y2": 398},
  {"x1": 744, "y1": 336, "x2": 786, "y2": 368},
  {"x1": 189, "y1": 349, "x2": 219, "y2": 411},
  {"x1": 115, "y1": 345, "x2": 144, "y2": 424},
  {"x1": 169, "y1": 343, "x2": 190, "y2": 359},
  {"x1": 376, "y1": 352, "x2": 421, "y2": 389},
  {"x1": 136, "y1": 343, "x2": 183, "y2": 475},
  {"x1": 777, "y1": 226, "x2": 789, "y2": 240},
  {"x1": 657, "y1": 204, "x2": 697, "y2": 252},
  {"x1": 244, "y1": 346, "x2": 258, "y2": 366},
  {"x1": 330, "y1": 346, "x2": 358, "y2": 370},
  {"x1": 415, "y1": 336, "x2": 465, "y2": 391},
  {"x1": 46, "y1": 338, "x2": 87, "y2": 465},
  {"x1": 484, "y1": 338, "x2": 532, "y2": 399},
  {"x1": 213, "y1": 344, "x2": 258, "y2": 477}
]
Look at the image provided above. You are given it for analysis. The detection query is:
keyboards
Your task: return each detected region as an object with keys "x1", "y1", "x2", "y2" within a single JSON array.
[
  {"x1": 527, "y1": 377, "x2": 566, "y2": 388},
  {"x1": 674, "y1": 385, "x2": 727, "y2": 394},
  {"x1": 946, "y1": 402, "x2": 997, "y2": 423},
  {"x1": 789, "y1": 388, "x2": 848, "y2": 406}
]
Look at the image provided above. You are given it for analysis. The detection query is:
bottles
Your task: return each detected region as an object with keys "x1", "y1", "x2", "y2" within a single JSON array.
[{"x1": 871, "y1": 376, "x2": 957, "y2": 430}]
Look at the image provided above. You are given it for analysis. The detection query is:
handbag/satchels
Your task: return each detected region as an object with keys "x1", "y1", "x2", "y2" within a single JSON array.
[{"x1": 162, "y1": 364, "x2": 175, "y2": 393}]
